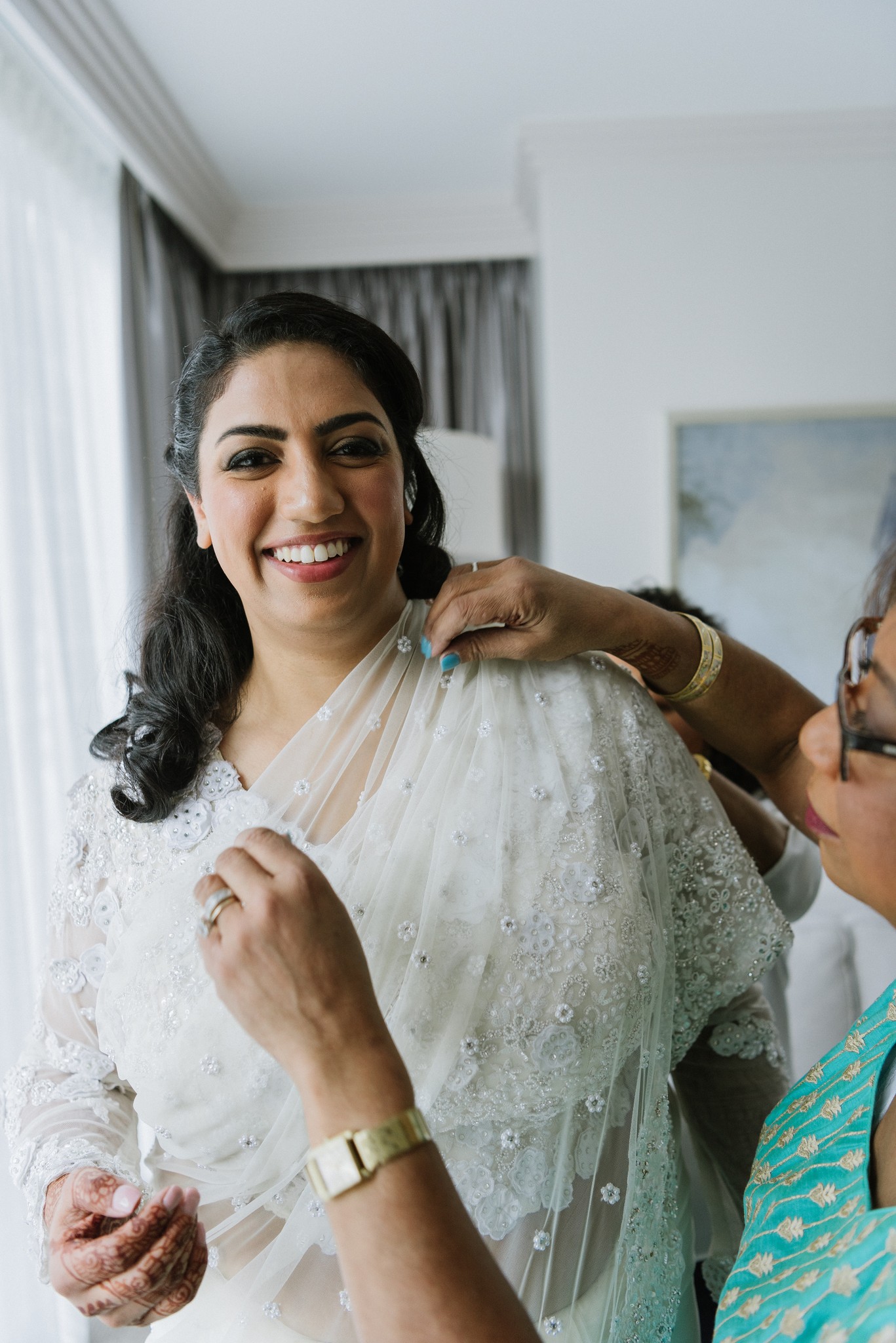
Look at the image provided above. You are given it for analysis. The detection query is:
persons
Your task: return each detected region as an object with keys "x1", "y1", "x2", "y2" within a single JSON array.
[
  {"x1": 20, "y1": 288, "x2": 796, "y2": 1341},
  {"x1": 595, "y1": 585, "x2": 824, "y2": 1342},
  {"x1": 186, "y1": 546, "x2": 896, "y2": 1343},
  {"x1": 756, "y1": 777, "x2": 896, "y2": 1079}
]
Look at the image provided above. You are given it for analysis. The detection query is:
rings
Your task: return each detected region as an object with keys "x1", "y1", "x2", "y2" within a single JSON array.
[
  {"x1": 471, "y1": 562, "x2": 477, "y2": 573},
  {"x1": 196, "y1": 886, "x2": 236, "y2": 936}
]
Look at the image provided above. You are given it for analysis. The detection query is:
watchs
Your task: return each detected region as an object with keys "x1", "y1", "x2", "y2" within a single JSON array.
[{"x1": 298, "y1": 1103, "x2": 433, "y2": 1205}]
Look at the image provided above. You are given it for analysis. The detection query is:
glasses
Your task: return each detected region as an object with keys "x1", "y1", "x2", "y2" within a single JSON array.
[{"x1": 837, "y1": 617, "x2": 896, "y2": 781}]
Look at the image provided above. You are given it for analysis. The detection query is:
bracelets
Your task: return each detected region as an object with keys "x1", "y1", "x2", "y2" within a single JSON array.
[
  {"x1": 690, "y1": 753, "x2": 711, "y2": 784},
  {"x1": 640, "y1": 611, "x2": 723, "y2": 703}
]
